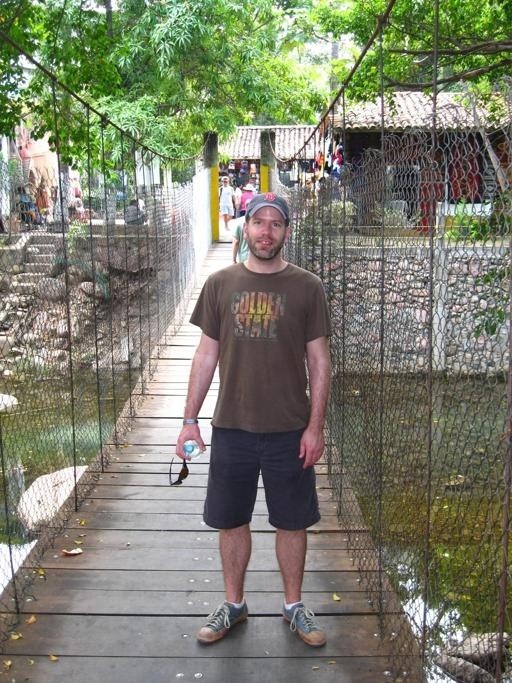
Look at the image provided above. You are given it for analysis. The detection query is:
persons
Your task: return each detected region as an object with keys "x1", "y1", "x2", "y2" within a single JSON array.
[
  {"x1": 176, "y1": 191, "x2": 343, "y2": 648},
  {"x1": 17, "y1": 166, "x2": 259, "y2": 232}
]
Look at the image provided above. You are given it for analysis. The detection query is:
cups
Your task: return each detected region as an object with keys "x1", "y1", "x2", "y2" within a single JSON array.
[{"x1": 169, "y1": 458, "x2": 189, "y2": 485}]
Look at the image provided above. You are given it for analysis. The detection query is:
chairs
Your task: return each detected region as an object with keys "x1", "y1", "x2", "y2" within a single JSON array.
[
  {"x1": 283, "y1": 599, "x2": 327, "y2": 648},
  {"x1": 197, "y1": 597, "x2": 249, "y2": 644},
  {"x1": 225, "y1": 226, "x2": 231, "y2": 232}
]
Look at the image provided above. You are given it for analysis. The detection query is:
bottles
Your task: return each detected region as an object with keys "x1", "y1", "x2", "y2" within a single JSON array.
[
  {"x1": 245, "y1": 193, "x2": 289, "y2": 221},
  {"x1": 222, "y1": 176, "x2": 229, "y2": 182},
  {"x1": 243, "y1": 184, "x2": 255, "y2": 191}
]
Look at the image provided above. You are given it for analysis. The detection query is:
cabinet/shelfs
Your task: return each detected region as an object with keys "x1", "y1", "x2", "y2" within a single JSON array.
[{"x1": 183, "y1": 440, "x2": 203, "y2": 461}]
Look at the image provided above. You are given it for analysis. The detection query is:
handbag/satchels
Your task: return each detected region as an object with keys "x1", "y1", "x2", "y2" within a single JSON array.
[{"x1": 182, "y1": 417, "x2": 198, "y2": 424}]
